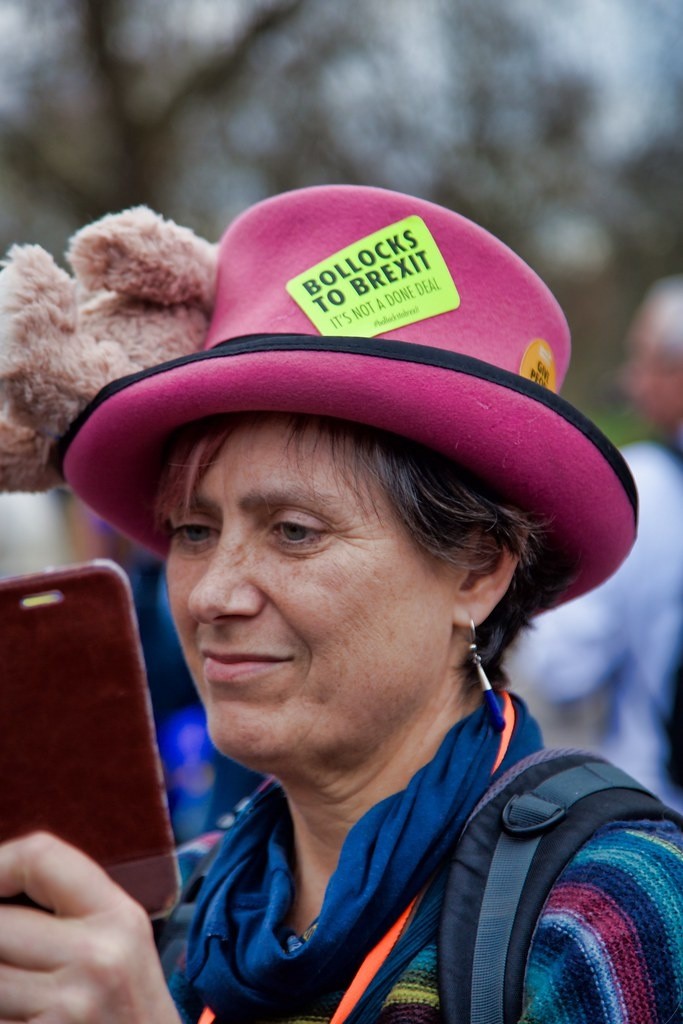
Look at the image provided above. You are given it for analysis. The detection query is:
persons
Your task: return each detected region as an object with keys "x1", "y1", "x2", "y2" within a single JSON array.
[{"x1": 1, "y1": 182, "x2": 682, "y2": 1023}]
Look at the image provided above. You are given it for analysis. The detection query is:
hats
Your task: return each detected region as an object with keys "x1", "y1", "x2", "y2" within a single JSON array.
[{"x1": 51, "y1": 185, "x2": 640, "y2": 617}]
